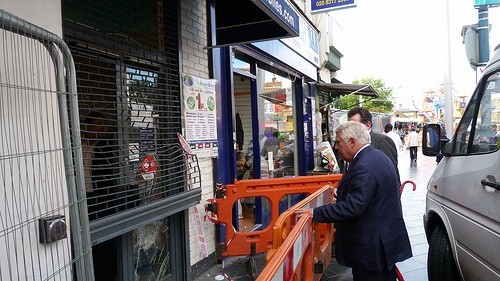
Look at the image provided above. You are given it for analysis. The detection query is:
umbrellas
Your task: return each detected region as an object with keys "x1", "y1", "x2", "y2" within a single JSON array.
[{"x1": 399, "y1": 180, "x2": 417, "y2": 196}]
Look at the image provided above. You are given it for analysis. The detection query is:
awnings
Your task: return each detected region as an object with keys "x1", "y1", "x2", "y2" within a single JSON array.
[
  {"x1": 258, "y1": 87, "x2": 293, "y2": 108},
  {"x1": 316, "y1": 82, "x2": 379, "y2": 114}
]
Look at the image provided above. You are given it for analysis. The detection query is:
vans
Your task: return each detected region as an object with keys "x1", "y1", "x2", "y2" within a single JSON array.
[{"x1": 419, "y1": 45, "x2": 500, "y2": 281}]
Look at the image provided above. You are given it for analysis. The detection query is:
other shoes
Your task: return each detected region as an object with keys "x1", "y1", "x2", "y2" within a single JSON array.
[{"x1": 411, "y1": 158, "x2": 417, "y2": 162}]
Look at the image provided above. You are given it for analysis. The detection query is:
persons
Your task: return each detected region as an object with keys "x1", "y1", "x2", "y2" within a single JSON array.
[
  {"x1": 235, "y1": 126, "x2": 294, "y2": 213},
  {"x1": 343, "y1": 107, "x2": 402, "y2": 197},
  {"x1": 382, "y1": 124, "x2": 402, "y2": 165},
  {"x1": 73, "y1": 111, "x2": 118, "y2": 274},
  {"x1": 407, "y1": 128, "x2": 421, "y2": 162},
  {"x1": 294, "y1": 121, "x2": 413, "y2": 281}
]
